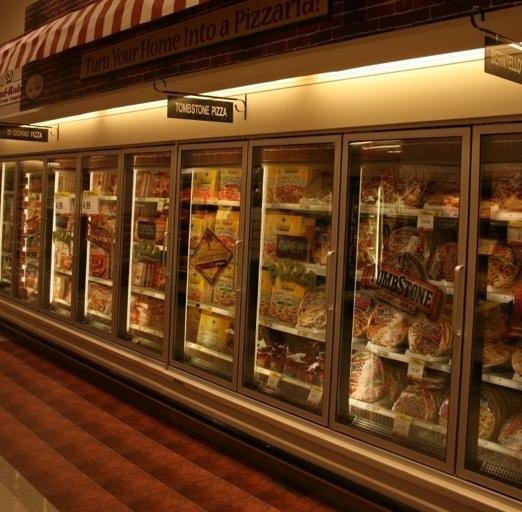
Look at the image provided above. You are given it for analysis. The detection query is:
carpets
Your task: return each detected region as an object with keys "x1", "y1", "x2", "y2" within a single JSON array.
[{"x1": 0, "y1": 340, "x2": 338, "y2": 512}]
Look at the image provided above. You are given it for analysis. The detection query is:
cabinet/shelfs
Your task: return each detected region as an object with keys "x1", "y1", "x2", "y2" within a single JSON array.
[{"x1": 0, "y1": 114, "x2": 522, "y2": 502}]
